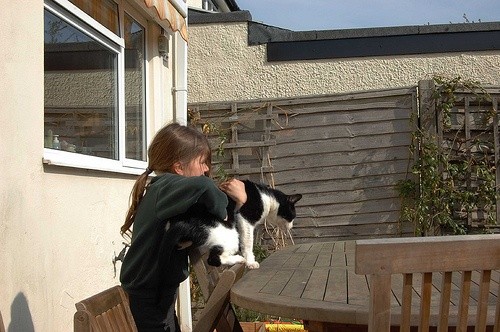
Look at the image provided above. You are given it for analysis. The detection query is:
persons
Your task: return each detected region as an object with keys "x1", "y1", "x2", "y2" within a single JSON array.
[{"x1": 118, "y1": 120, "x2": 249, "y2": 332}]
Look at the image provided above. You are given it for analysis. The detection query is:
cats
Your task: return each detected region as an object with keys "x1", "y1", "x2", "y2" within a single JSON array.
[{"x1": 164, "y1": 180, "x2": 303, "y2": 270}]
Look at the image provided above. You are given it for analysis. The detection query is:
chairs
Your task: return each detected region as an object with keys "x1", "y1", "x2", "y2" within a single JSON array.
[
  {"x1": 73, "y1": 263, "x2": 245, "y2": 332},
  {"x1": 353, "y1": 235, "x2": 500, "y2": 332}
]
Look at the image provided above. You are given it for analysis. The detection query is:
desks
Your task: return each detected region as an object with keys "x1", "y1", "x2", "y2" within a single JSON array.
[{"x1": 231, "y1": 238, "x2": 500, "y2": 332}]
